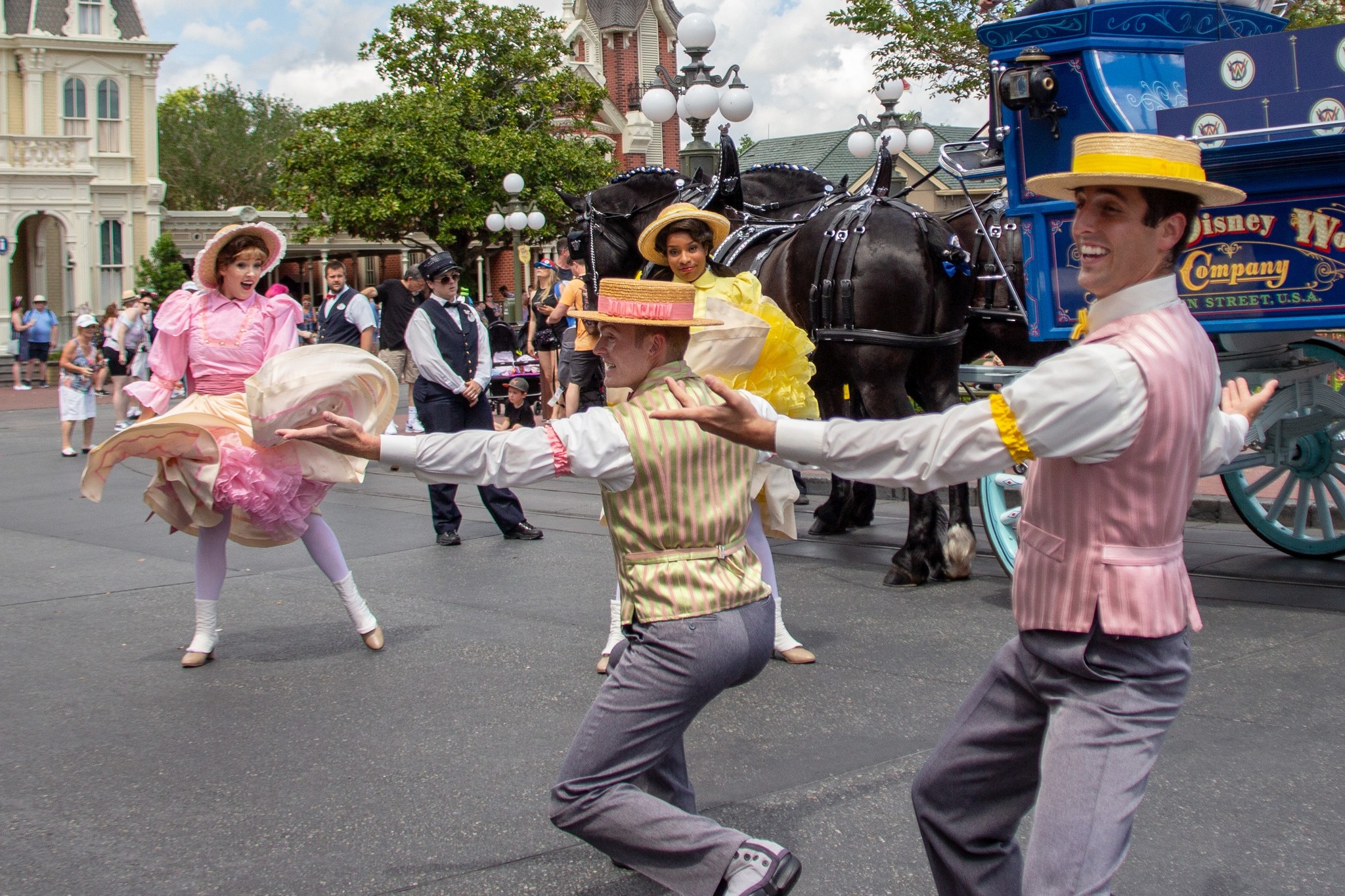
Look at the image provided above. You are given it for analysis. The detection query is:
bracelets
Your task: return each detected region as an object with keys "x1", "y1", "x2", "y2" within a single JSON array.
[{"x1": 526, "y1": 340, "x2": 533, "y2": 342}]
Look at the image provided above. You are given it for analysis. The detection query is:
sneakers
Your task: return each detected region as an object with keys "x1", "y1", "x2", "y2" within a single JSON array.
[
  {"x1": 436, "y1": 528, "x2": 461, "y2": 546},
  {"x1": 503, "y1": 519, "x2": 544, "y2": 540},
  {"x1": 383, "y1": 417, "x2": 425, "y2": 435}
]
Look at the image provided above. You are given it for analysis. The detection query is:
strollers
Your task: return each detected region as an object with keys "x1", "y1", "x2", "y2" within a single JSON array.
[{"x1": 486, "y1": 321, "x2": 543, "y2": 417}]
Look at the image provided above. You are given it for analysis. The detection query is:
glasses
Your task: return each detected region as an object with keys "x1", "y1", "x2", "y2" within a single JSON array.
[
  {"x1": 303, "y1": 302, "x2": 311, "y2": 305},
  {"x1": 432, "y1": 272, "x2": 460, "y2": 286},
  {"x1": 486, "y1": 295, "x2": 493, "y2": 298},
  {"x1": 138, "y1": 301, "x2": 153, "y2": 308},
  {"x1": 536, "y1": 265, "x2": 550, "y2": 269}
]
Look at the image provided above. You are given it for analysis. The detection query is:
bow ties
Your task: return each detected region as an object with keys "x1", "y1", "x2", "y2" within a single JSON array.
[
  {"x1": 950, "y1": 233, "x2": 971, "y2": 263},
  {"x1": 325, "y1": 294, "x2": 336, "y2": 300},
  {"x1": 444, "y1": 301, "x2": 461, "y2": 308},
  {"x1": 1072, "y1": 308, "x2": 1089, "y2": 340},
  {"x1": 943, "y1": 260, "x2": 971, "y2": 278}
]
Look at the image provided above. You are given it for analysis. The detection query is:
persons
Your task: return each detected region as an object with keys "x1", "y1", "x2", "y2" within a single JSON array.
[
  {"x1": 494, "y1": 378, "x2": 536, "y2": 430},
  {"x1": 599, "y1": 204, "x2": 817, "y2": 673},
  {"x1": 651, "y1": 131, "x2": 1279, "y2": 896},
  {"x1": 9, "y1": 295, "x2": 60, "y2": 391},
  {"x1": 457, "y1": 284, "x2": 535, "y2": 324},
  {"x1": 276, "y1": 277, "x2": 803, "y2": 896},
  {"x1": 367, "y1": 297, "x2": 382, "y2": 354},
  {"x1": 77, "y1": 219, "x2": 383, "y2": 666},
  {"x1": 361, "y1": 263, "x2": 425, "y2": 435},
  {"x1": 264, "y1": 283, "x2": 319, "y2": 346},
  {"x1": 102, "y1": 294, "x2": 153, "y2": 432},
  {"x1": 319, "y1": 261, "x2": 378, "y2": 354},
  {"x1": 404, "y1": 253, "x2": 546, "y2": 544},
  {"x1": 92, "y1": 281, "x2": 197, "y2": 419},
  {"x1": 57, "y1": 315, "x2": 100, "y2": 456},
  {"x1": 526, "y1": 237, "x2": 600, "y2": 419}
]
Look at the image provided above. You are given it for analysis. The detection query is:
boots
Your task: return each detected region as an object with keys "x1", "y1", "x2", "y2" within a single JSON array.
[
  {"x1": 333, "y1": 570, "x2": 384, "y2": 650},
  {"x1": 596, "y1": 598, "x2": 625, "y2": 673},
  {"x1": 771, "y1": 597, "x2": 816, "y2": 664},
  {"x1": 181, "y1": 598, "x2": 219, "y2": 667}
]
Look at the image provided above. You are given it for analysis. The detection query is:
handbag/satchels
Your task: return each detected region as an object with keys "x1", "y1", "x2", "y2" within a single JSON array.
[
  {"x1": 534, "y1": 329, "x2": 556, "y2": 348},
  {"x1": 91, "y1": 325, "x2": 106, "y2": 348},
  {"x1": 7, "y1": 339, "x2": 20, "y2": 355}
]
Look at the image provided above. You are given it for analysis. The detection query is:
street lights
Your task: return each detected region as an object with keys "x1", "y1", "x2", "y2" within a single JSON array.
[
  {"x1": 487, "y1": 172, "x2": 547, "y2": 327},
  {"x1": 847, "y1": 69, "x2": 936, "y2": 205},
  {"x1": 641, "y1": 8, "x2": 754, "y2": 181}
]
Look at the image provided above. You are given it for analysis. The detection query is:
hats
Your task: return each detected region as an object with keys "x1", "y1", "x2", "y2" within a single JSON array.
[
  {"x1": 33, "y1": 221, "x2": 289, "y2": 328},
  {"x1": 418, "y1": 250, "x2": 461, "y2": 282},
  {"x1": 534, "y1": 258, "x2": 556, "y2": 271},
  {"x1": 502, "y1": 378, "x2": 529, "y2": 393},
  {"x1": 567, "y1": 202, "x2": 731, "y2": 327},
  {"x1": 1026, "y1": 132, "x2": 1247, "y2": 208}
]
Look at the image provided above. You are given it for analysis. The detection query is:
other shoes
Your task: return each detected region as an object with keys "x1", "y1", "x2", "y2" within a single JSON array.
[
  {"x1": 793, "y1": 493, "x2": 810, "y2": 505},
  {"x1": 723, "y1": 838, "x2": 802, "y2": 896},
  {"x1": 13, "y1": 381, "x2": 186, "y2": 456}
]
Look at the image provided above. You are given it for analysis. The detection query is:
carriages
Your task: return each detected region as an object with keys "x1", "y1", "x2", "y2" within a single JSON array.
[{"x1": 554, "y1": 1, "x2": 1343, "y2": 589}]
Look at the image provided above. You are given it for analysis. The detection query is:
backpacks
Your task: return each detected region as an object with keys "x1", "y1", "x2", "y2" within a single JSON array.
[{"x1": 555, "y1": 277, "x2": 603, "y2": 393}]
